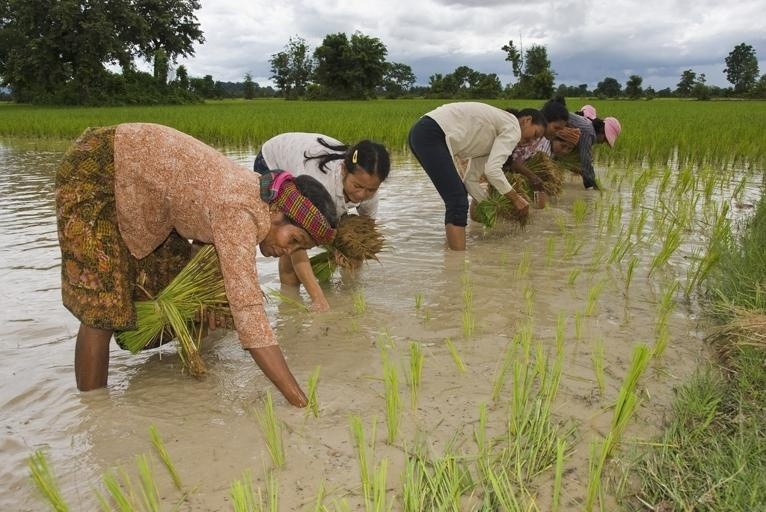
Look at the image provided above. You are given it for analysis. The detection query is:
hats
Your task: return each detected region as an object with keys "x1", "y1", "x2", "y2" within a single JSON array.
[
  {"x1": 580, "y1": 104, "x2": 596, "y2": 120},
  {"x1": 602, "y1": 116, "x2": 621, "y2": 149}
]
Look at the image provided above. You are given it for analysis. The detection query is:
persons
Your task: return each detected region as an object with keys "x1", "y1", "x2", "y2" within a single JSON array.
[
  {"x1": 408, "y1": 95, "x2": 621, "y2": 251},
  {"x1": 254, "y1": 132, "x2": 390, "y2": 309},
  {"x1": 57, "y1": 123, "x2": 338, "y2": 408}
]
